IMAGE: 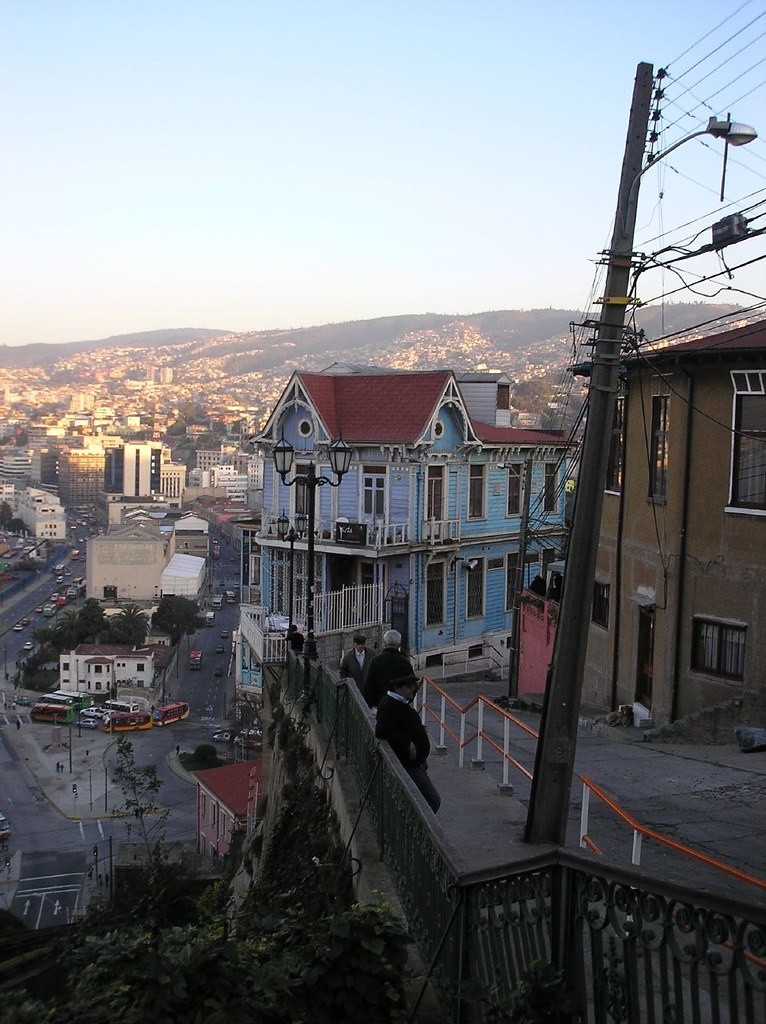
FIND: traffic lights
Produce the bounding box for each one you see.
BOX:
[72,784,77,793]
[92,846,98,857]
[4,857,10,868]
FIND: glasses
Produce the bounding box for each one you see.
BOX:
[354,643,365,645]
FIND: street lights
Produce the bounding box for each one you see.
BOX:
[272,427,353,659]
[78,690,84,738]
[525,116,758,847]
[497,458,532,700]
[276,508,307,629]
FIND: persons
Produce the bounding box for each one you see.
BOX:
[375,666,442,814]
[531,573,547,598]
[56,762,64,773]
[4,700,16,710]
[285,625,305,657]
[16,720,20,730]
[176,745,180,755]
[339,636,377,682]
[361,630,414,708]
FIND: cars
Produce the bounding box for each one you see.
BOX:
[0,813,12,839]
[3,538,35,558]
[213,727,259,747]
[13,564,85,650]
[70,514,104,562]
[77,719,98,729]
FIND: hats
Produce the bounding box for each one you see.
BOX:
[387,668,420,687]
[353,635,366,642]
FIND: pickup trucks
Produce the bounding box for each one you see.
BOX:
[80,708,106,718]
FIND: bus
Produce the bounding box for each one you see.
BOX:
[99,700,140,714]
[152,702,190,726]
[103,712,152,731]
[30,690,94,723]
[188,581,239,676]
